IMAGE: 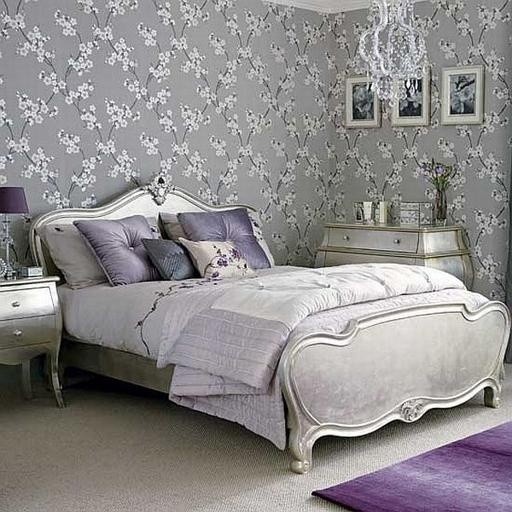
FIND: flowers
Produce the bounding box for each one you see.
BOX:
[420,159,460,218]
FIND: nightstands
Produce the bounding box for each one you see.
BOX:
[0,273,66,411]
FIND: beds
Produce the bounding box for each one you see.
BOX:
[30,172,512,475]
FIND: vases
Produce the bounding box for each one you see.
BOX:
[435,189,448,224]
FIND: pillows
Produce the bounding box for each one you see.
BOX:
[178,209,270,268]
[159,211,274,264]
[142,237,192,280]
[178,234,253,278]
[74,215,158,285]
[37,217,162,289]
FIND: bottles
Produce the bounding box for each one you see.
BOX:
[354,202,363,223]
[374,204,380,222]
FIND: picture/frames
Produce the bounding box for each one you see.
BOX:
[442,65,484,124]
[344,76,381,128]
[392,67,430,128]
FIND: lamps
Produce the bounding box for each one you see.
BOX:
[0,188,29,275]
[356,0,427,118]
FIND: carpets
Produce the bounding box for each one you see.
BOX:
[311,420,512,512]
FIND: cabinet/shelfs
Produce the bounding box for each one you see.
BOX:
[315,222,473,290]
